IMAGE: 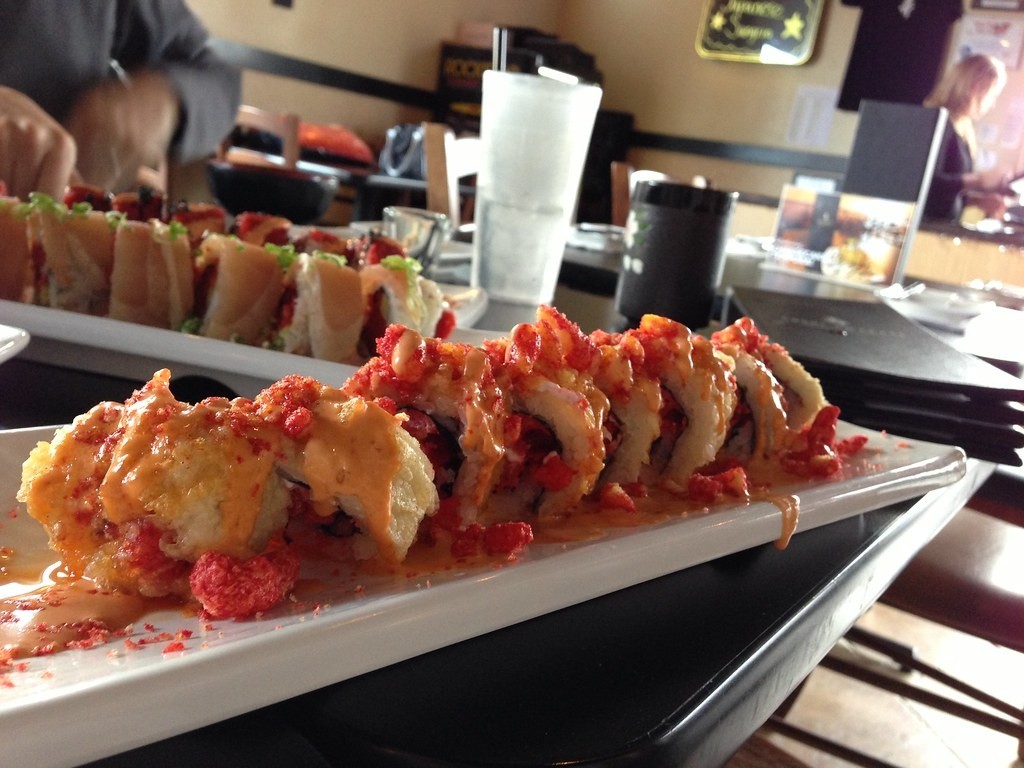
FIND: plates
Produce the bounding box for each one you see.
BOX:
[893,301,984,325]
[0,418,971,768]
[0,298,362,399]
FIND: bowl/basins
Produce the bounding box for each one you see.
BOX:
[205,160,340,224]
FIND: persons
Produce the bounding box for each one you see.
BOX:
[918,54,1024,226]
[1,0,243,213]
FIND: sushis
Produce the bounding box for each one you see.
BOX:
[18,300,824,604]
[1,184,455,367]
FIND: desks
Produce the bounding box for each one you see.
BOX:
[211,146,1024,504]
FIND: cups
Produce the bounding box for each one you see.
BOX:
[470,69,603,305]
[614,179,740,330]
[381,205,454,282]
